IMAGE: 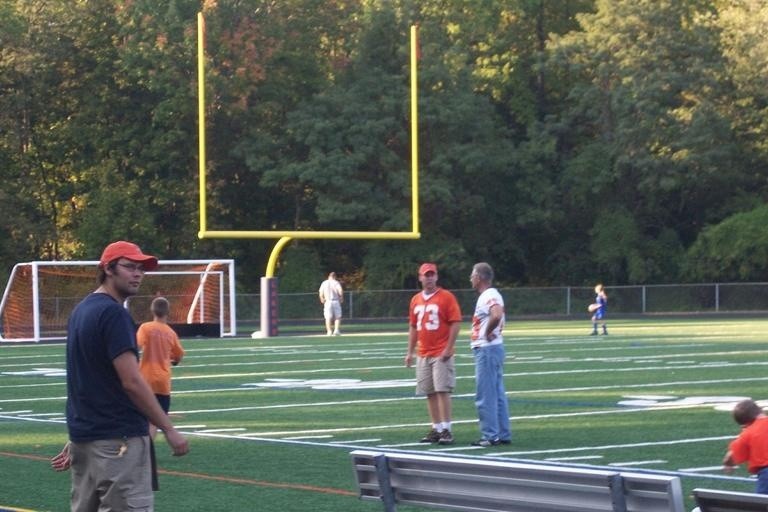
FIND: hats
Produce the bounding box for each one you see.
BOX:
[418,263,437,275]
[101,241,158,271]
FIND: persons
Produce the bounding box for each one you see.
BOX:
[319,272,344,335]
[51,240,189,512]
[589,285,608,335]
[136,297,186,440]
[470,263,511,446]
[405,263,462,443]
[723,401,768,494]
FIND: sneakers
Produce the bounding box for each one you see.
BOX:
[439,429,454,442]
[472,438,512,446]
[420,429,441,442]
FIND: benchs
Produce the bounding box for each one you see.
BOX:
[691,486,767,512]
[346,446,686,512]
